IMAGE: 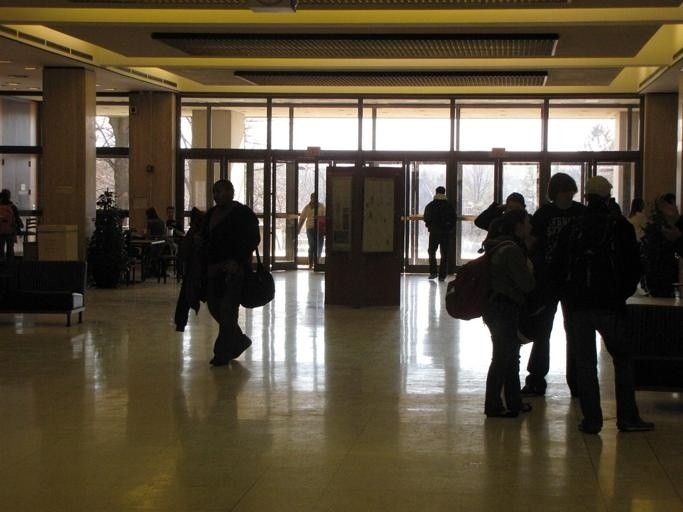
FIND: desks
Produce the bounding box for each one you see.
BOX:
[121,237,165,289]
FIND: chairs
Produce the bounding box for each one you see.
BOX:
[159,239,188,284]
[21,217,39,256]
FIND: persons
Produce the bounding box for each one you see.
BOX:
[192,179,261,366]
[623,197,652,296]
[516,173,587,398]
[556,175,657,433]
[474,192,534,261]
[298,192,326,268]
[141,208,165,258]
[654,193,682,296]
[0,189,23,257]
[423,187,457,281]
[477,209,533,418]
[165,205,183,280]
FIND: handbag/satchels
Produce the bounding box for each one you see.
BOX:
[239,242,275,310]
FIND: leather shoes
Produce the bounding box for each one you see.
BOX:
[428,273,437,279]
[439,276,445,281]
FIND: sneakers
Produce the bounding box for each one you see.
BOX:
[519,386,545,398]
[577,422,604,434]
[617,418,656,432]
[208,333,251,366]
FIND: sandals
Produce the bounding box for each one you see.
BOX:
[485,399,534,418]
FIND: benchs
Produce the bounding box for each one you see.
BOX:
[0,257,90,328]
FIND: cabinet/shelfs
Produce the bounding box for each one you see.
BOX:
[37,223,79,262]
[614,290,683,395]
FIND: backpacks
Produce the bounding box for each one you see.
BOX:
[445,240,517,321]
[0,203,15,232]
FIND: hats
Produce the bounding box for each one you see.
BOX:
[583,176,612,197]
